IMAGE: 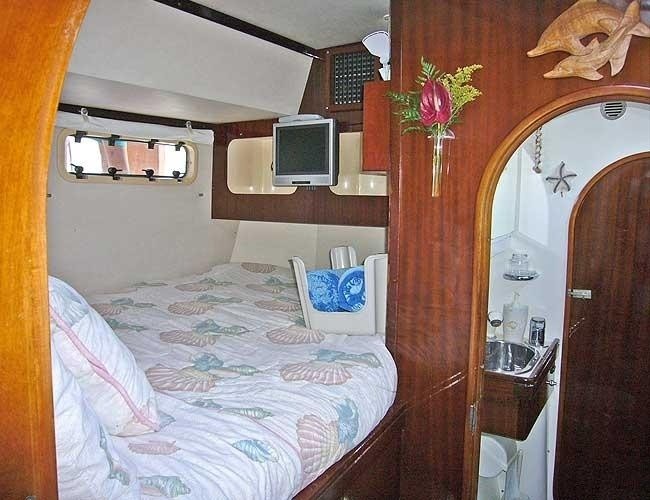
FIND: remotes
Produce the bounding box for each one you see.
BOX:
[279,114,324,122]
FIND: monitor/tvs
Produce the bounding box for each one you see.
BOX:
[273,118,339,186]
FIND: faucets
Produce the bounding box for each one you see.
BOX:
[487,310,504,339]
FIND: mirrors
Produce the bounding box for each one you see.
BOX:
[63,135,191,183]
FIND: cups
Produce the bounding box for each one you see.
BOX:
[510,253,530,276]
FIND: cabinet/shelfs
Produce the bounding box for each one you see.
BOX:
[483,362,556,441]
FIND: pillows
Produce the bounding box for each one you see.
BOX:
[48,274,173,438]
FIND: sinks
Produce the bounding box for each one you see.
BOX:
[483,339,541,374]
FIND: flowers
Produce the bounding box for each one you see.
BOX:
[382,59,482,196]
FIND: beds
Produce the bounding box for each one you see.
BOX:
[53,259,397,492]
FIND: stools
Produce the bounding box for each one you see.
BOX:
[290,245,388,336]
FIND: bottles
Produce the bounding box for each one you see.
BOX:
[530,316,546,347]
[501,290,530,344]
